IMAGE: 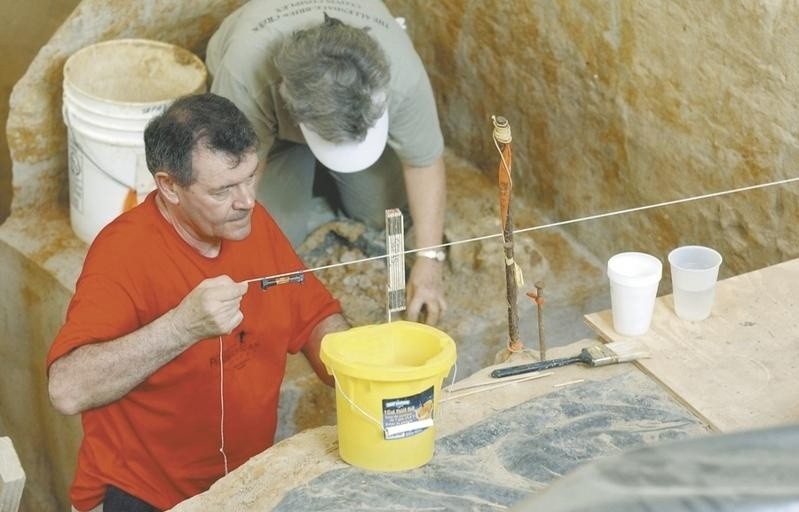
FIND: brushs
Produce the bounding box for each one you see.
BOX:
[491,340,652,378]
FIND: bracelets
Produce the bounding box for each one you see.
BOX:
[416,249,448,263]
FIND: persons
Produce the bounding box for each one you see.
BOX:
[204,1,452,329]
[45,90,353,511]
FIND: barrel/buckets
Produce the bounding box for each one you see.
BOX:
[62,39,209,245]
[318,319,458,471]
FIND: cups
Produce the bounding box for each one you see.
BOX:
[668,245,723,322]
[606,252,663,336]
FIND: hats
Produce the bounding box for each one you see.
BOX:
[298,87,390,175]
